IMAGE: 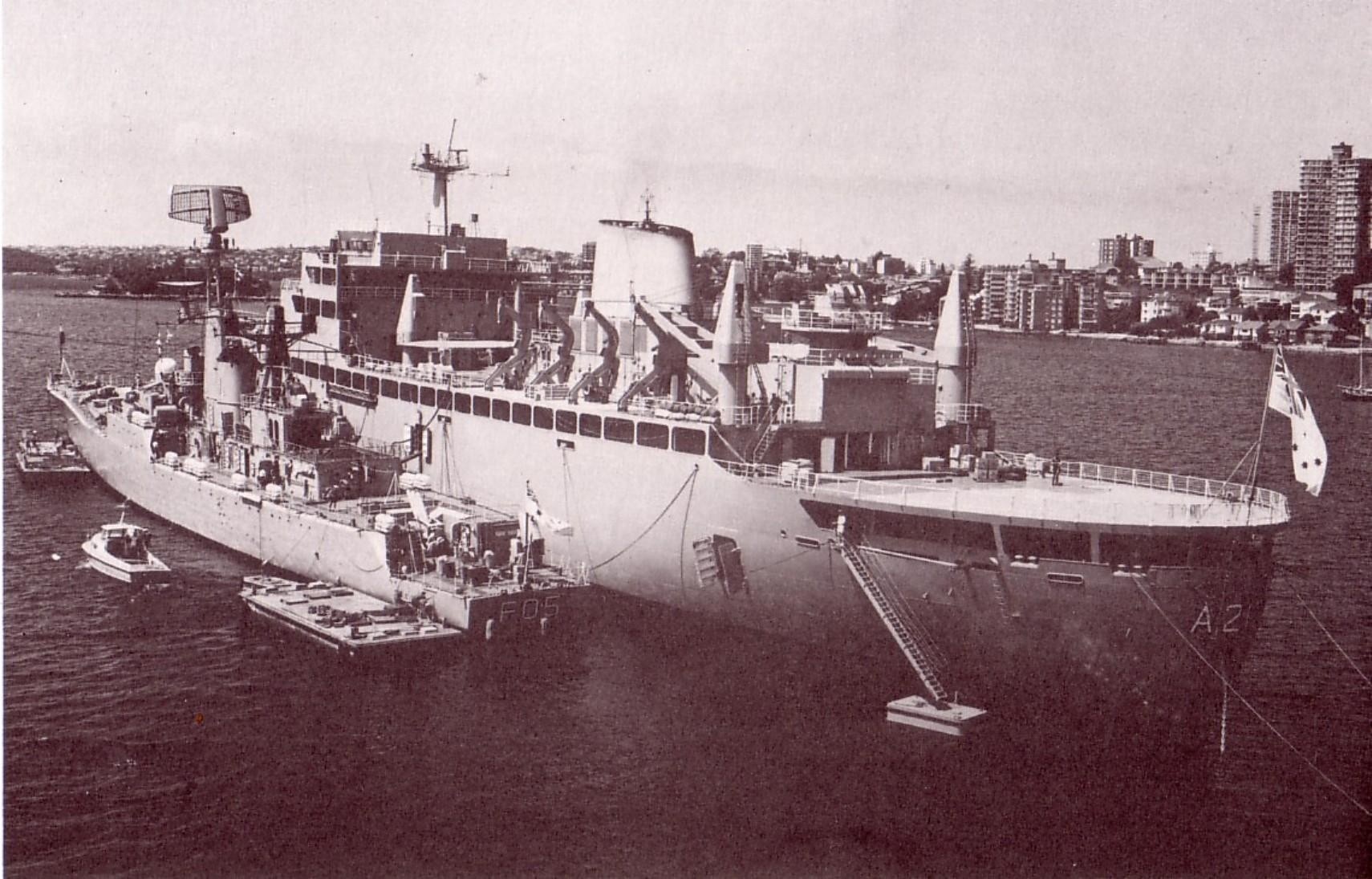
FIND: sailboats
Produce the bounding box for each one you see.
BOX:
[1335,325,1372,400]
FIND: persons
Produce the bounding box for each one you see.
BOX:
[257,451,293,490]
[121,526,153,563]
[1051,451,1061,485]
[130,372,143,392]
[325,485,340,508]
[746,392,784,422]
[23,426,67,458]
[504,370,522,392]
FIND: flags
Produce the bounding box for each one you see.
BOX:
[526,488,544,520]
[1266,343,1328,497]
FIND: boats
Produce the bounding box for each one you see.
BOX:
[14,427,91,487]
[45,184,599,641]
[239,573,465,661]
[82,499,173,587]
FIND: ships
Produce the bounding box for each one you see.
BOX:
[242,117,1294,746]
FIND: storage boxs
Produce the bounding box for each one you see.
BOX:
[922,443,997,481]
